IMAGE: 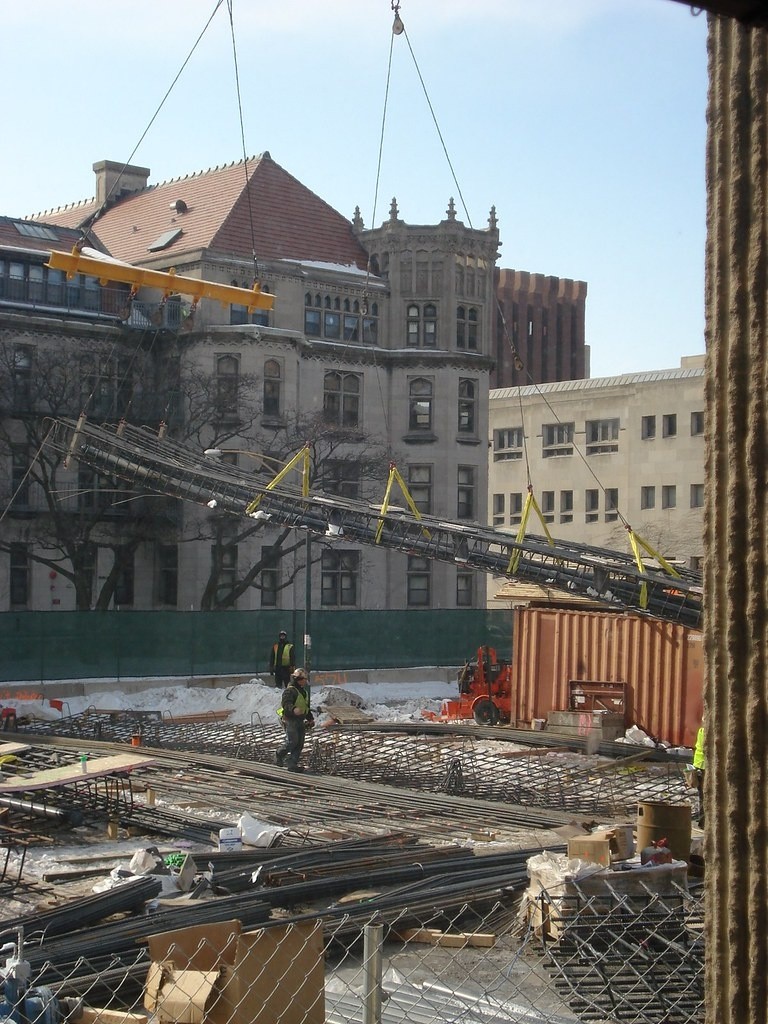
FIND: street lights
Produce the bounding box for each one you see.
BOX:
[204,439,314,713]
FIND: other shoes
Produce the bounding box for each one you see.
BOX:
[276,751,283,767]
[288,765,303,773]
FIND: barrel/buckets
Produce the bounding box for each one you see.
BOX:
[634,800,692,874]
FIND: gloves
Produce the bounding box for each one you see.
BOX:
[270,667,275,675]
[289,665,295,674]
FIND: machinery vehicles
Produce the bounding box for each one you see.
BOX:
[418,642,514,730]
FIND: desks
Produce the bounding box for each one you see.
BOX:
[0,754,156,824]
[0,742,32,753]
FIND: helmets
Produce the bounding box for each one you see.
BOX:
[290,668,309,679]
[278,631,288,636]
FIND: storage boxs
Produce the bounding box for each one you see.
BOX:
[532,718,546,730]
[146,919,325,1024]
[548,679,626,742]
[527,824,686,941]
[161,852,197,893]
[210,827,242,852]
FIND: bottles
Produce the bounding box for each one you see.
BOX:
[81,757,88,774]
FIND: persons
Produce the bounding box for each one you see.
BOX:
[275,668,315,772]
[270,631,296,688]
[692,728,704,822]
